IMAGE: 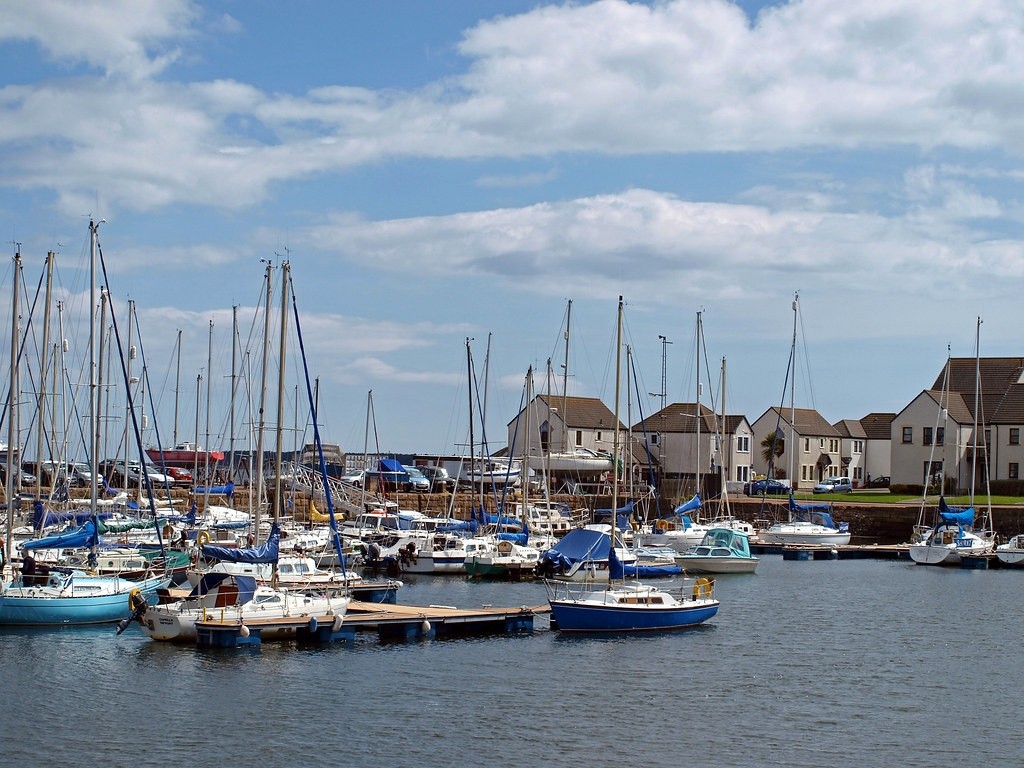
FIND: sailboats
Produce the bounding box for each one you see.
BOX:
[1,215,1023,651]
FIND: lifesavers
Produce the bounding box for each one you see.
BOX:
[198,531,210,545]
[656,519,668,530]
[693,577,712,599]
[129,588,141,611]
